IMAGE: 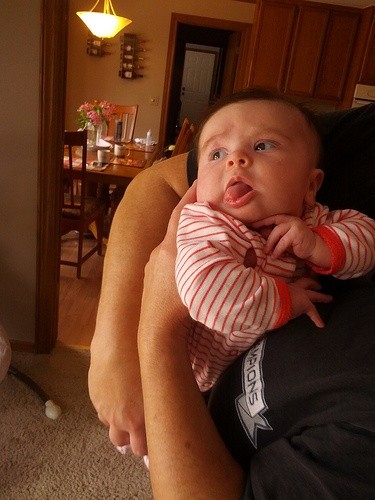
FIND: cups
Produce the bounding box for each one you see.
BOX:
[114,144,130,158]
[97,150,110,162]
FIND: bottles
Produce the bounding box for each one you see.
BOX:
[146,129,152,146]
[86,122,95,151]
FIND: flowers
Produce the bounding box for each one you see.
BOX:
[76,99,116,131]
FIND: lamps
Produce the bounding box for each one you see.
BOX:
[76,0,133,39]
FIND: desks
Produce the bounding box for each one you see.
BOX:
[61,137,162,236]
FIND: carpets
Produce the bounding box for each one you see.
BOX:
[0,343,153,500]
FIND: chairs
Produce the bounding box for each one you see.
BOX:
[104,103,138,140]
[169,119,196,156]
[60,130,108,279]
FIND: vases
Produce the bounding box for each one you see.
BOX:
[87,121,108,150]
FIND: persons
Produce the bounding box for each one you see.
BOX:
[108,88,375,471]
[87,100,375,500]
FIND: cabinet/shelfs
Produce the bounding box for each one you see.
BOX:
[119,33,137,78]
[87,31,104,57]
[243,0,375,109]
